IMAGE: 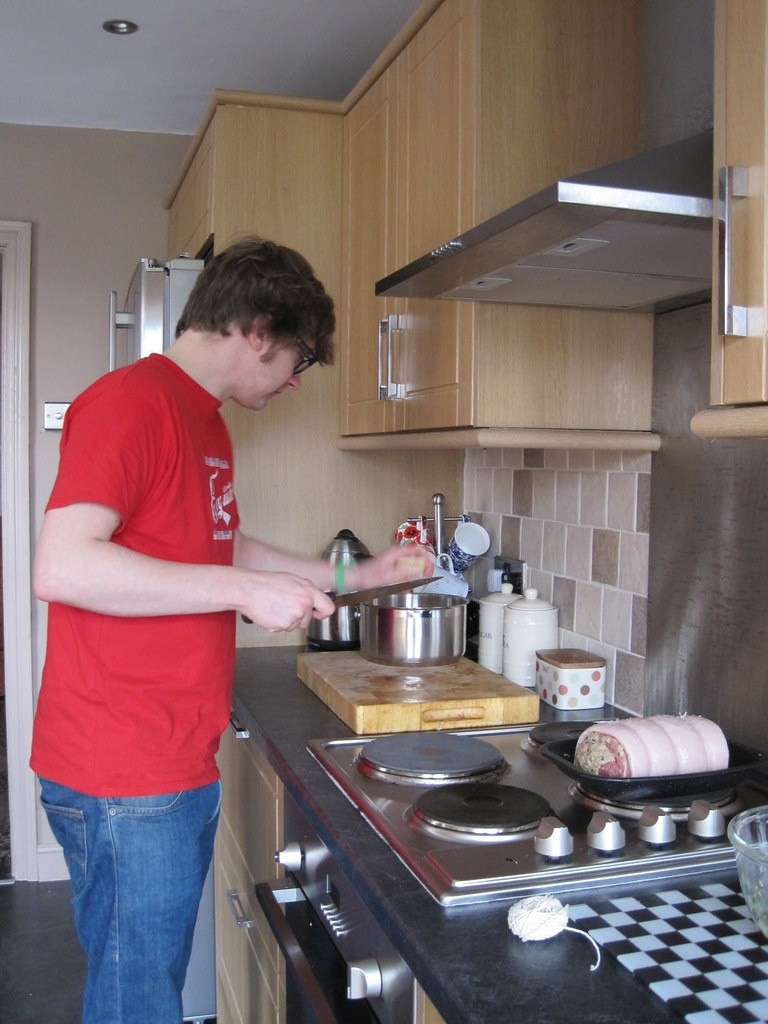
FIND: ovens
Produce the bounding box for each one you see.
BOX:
[256,783,420,1024]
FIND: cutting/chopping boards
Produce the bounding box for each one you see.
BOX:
[295,651,539,735]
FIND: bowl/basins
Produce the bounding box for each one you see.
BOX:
[726,805,768,940]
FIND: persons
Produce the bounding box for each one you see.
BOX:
[30,238,434,1024]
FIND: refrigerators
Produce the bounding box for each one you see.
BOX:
[107,252,215,1024]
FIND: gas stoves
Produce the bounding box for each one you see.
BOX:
[305,713,768,907]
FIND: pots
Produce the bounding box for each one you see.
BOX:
[351,592,471,667]
[541,739,768,801]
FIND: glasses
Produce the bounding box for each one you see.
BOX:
[288,331,319,375]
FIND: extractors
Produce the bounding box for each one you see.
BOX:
[375,128,725,315]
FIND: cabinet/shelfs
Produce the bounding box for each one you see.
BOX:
[691,0,768,441]
[215,705,285,1023]
[163,1,664,452]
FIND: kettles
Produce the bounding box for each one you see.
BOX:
[306,530,376,651]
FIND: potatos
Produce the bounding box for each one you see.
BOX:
[393,555,426,577]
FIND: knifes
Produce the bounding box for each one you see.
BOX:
[241,575,444,626]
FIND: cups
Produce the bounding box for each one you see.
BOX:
[503,588,559,686]
[478,582,524,674]
[445,515,491,572]
[395,514,436,556]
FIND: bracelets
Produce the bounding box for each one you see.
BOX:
[336,564,345,592]
